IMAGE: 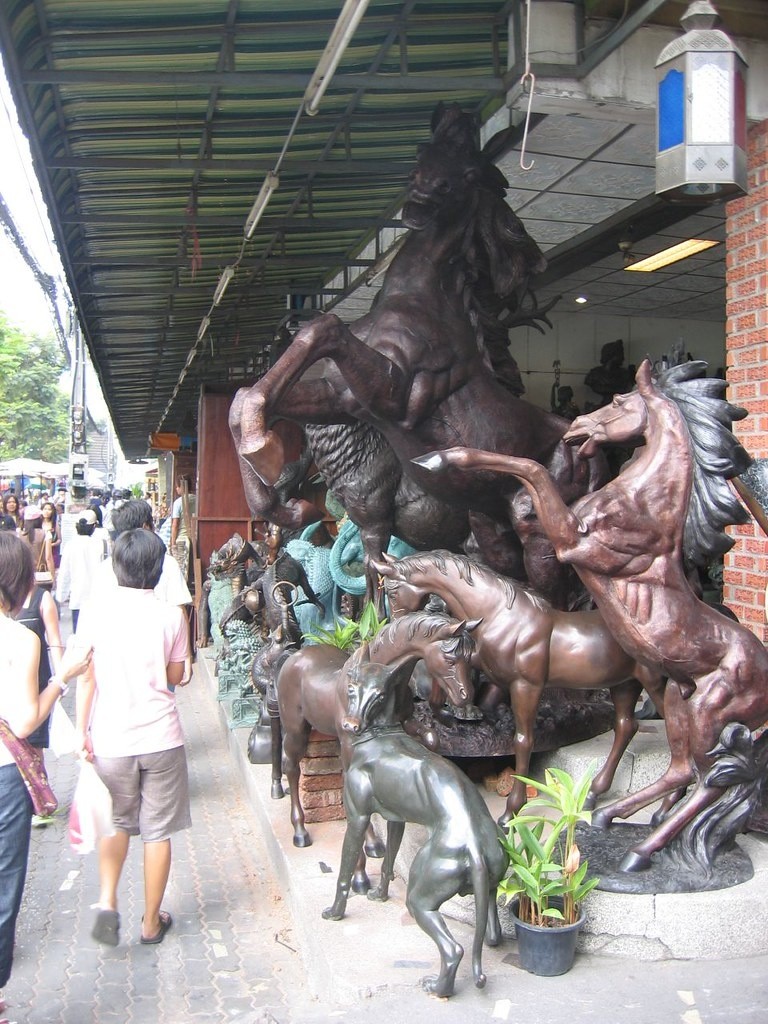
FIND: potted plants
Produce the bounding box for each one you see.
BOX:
[492,756,600,974]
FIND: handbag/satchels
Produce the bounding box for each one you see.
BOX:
[9,737,58,817]
[33,563,54,585]
[69,749,116,855]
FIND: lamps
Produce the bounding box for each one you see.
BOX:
[654,0,751,208]
[155,369,187,433]
[303,0,371,116]
[186,349,197,366]
[197,316,211,340]
[212,265,238,307]
[242,170,281,241]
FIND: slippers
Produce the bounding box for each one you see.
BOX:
[140,909,171,944]
[92,911,120,947]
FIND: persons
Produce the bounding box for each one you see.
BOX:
[0,472,197,988]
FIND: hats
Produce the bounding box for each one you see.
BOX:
[76,509,100,525]
[24,505,44,520]
[115,491,123,497]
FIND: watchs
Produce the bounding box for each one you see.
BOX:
[48,675,70,697]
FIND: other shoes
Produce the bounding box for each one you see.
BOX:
[0,990,5,1013]
[31,814,55,827]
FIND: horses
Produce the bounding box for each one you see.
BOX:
[207,97,768,998]
